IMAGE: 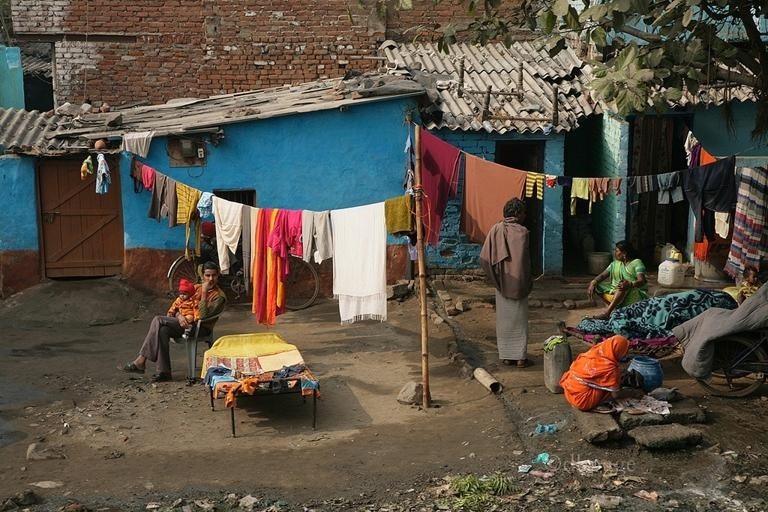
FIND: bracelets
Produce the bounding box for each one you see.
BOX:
[629,281,634,287]
[590,280,598,287]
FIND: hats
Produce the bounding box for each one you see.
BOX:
[179,279,195,296]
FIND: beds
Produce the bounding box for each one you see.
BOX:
[558,321,679,367]
[204,333,320,437]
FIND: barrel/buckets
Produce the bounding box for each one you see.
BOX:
[543,341,571,394]
[655,241,685,288]
[587,251,611,275]
[627,356,664,395]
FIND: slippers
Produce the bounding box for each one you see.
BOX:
[117,362,144,374]
[592,401,617,414]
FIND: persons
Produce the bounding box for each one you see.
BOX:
[482,197,536,369]
[112,261,228,381]
[166,279,201,339]
[737,262,762,306]
[587,240,649,320]
[557,335,646,413]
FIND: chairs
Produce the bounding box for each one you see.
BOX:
[170,308,225,380]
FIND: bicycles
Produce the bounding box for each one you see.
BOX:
[687,326,767,399]
[166,221,320,311]
